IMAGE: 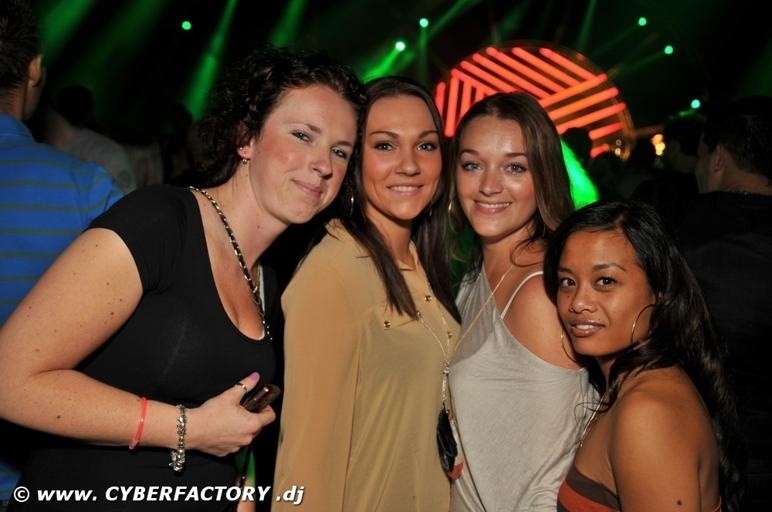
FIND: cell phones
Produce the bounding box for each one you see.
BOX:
[243,383,281,414]
